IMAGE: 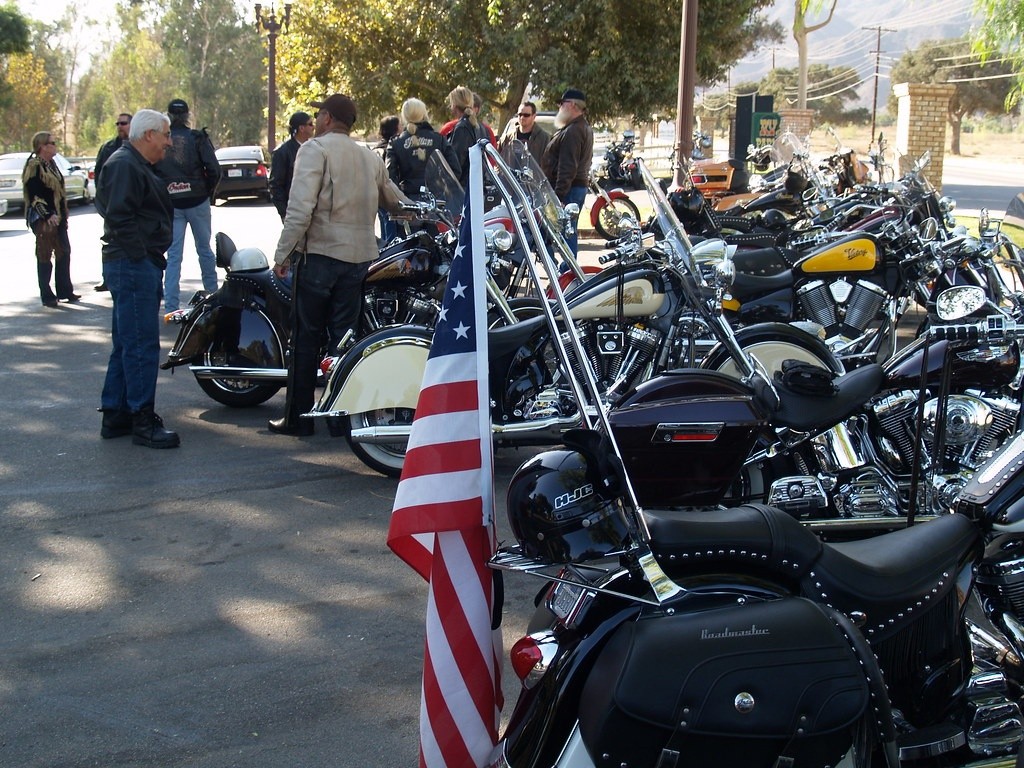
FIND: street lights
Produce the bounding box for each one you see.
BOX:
[252,1,294,165]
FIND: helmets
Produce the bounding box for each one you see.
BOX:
[667,187,704,228]
[623,130,635,137]
[507,449,627,562]
[701,139,711,148]
[230,247,269,273]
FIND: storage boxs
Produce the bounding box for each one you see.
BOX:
[589,367,764,509]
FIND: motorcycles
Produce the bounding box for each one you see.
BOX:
[668,129,712,183]
[602,129,644,187]
[156,146,468,410]
[473,122,1024,768]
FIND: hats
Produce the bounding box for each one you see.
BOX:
[554,90,585,104]
[310,93,357,124]
[168,99,188,114]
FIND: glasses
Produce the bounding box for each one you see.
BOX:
[560,101,576,106]
[115,122,130,126]
[45,142,55,145]
[313,112,327,118]
[518,113,533,117]
[304,122,314,126]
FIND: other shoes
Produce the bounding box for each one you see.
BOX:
[58,294,81,301]
[43,301,56,307]
[95,284,109,291]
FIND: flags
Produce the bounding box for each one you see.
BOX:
[385,194,509,768]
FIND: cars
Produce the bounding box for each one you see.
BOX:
[0,152,96,217]
[206,146,273,206]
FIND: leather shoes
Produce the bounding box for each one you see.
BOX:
[101,410,134,437]
[328,417,347,436]
[268,417,314,435]
[132,409,180,448]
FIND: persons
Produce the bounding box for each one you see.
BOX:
[93,110,181,448]
[541,88,594,274]
[267,112,316,225]
[448,85,491,184]
[438,92,496,167]
[154,98,221,315]
[384,98,462,267]
[269,94,417,435]
[92,113,132,290]
[21,131,81,308]
[498,100,549,207]
[372,116,402,242]
